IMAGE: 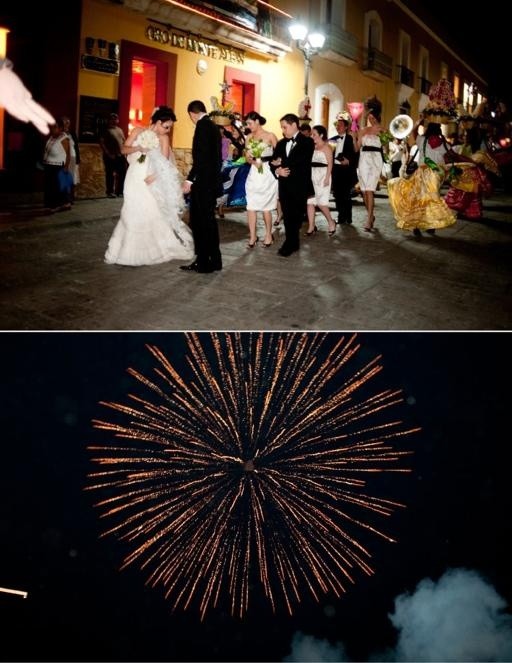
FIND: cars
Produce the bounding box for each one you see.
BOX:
[487,137,511,151]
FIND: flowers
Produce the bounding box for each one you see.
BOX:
[374,126,394,162]
[247,135,272,173]
[137,128,161,162]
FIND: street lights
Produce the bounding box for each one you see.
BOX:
[287,12,328,129]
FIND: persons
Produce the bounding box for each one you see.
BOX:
[44,120,81,218]
[59,116,81,161]
[102,96,494,269]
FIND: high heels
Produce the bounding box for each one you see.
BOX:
[249,215,317,256]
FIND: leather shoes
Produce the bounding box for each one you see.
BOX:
[180,255,222,273]
[328,216,375,237]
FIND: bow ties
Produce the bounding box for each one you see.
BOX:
[337,136,343,139]
[286,137,295,143]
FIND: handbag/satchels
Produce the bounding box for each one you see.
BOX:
[406,162,418,175]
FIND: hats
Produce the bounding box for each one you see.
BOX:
[460,121,473,129]
[479,123,488,128]
[212,116,232,126]
[424,114,452,124]
